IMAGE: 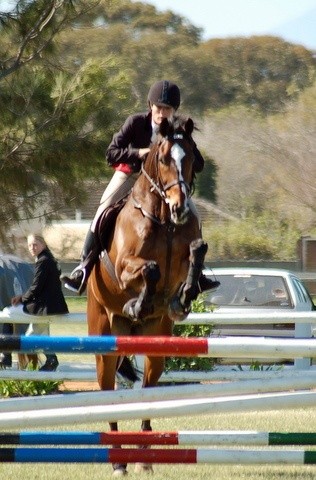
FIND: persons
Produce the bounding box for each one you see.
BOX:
[61,86,221,294]
[11,231,70,373]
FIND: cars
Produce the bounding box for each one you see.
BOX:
[131,266,316,370]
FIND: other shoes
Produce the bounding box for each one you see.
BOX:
[60,272,86,296]
[39,354,58,372]
[0,353,12,367]
[195,275,220,291]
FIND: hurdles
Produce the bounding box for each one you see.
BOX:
[0,310,316,466]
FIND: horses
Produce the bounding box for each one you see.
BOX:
[87,117,208,477]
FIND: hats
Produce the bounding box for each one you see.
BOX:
[147,80,181,112]
